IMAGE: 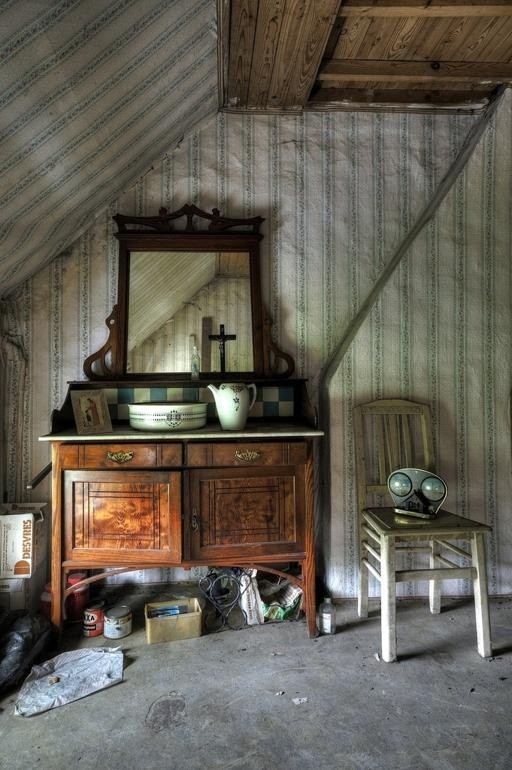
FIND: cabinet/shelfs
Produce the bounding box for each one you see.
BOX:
[38,427,326,642]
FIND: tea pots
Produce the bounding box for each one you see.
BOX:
[208,382,258,433]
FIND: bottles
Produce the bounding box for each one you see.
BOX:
[318,596,336,635]
[39,571,92,624]
[191,347,201,381]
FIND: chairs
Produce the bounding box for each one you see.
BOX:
[348,397,495,664]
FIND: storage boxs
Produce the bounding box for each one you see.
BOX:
[0,500,49,581]
[1,562,47,618]
[145,593,205,646]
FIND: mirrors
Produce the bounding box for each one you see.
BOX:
[80,203,295,381]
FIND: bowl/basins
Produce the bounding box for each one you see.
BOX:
[126,400,211,431]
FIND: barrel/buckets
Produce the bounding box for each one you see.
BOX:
[40,583,52,620]
[82,600,106,638]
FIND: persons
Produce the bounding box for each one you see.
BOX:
[85,398,100,426]
